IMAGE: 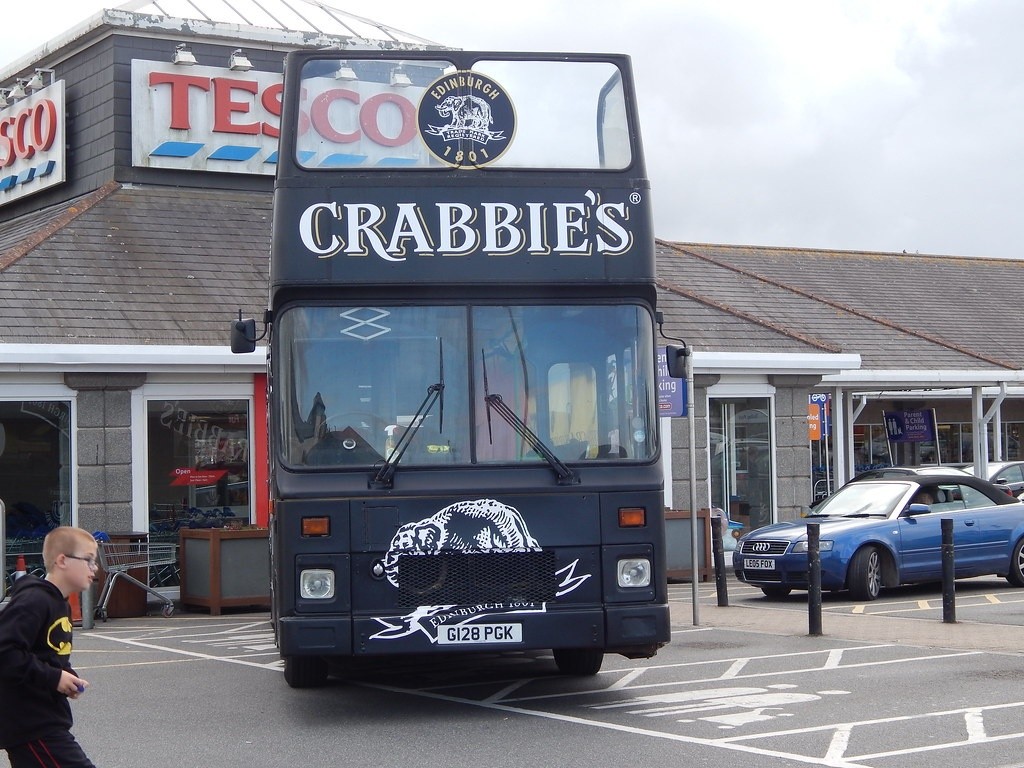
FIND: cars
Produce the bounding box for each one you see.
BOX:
[962,461,1024,498]
[709,409,1022,528]
[807,465,1012,510]
[733,466,1024,602]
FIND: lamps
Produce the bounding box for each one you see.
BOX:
[228,48,254,72]
[171,41,198,66]
[0,68,56,107]
[390,66,413,88]
[335,59,357,82]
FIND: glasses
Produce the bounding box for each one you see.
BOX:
[64,553,98,565]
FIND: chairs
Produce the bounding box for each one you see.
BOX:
[932,489,946,504]
[942,489,953,502]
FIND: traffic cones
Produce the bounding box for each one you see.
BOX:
[16,553,27,583]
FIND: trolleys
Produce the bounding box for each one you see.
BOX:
[6,506,241,594]
[92,531,179,623]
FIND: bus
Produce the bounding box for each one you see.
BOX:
[226,45,693,689]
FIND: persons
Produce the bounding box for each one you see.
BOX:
[0,526,98,768]
[917,493,933,508]
